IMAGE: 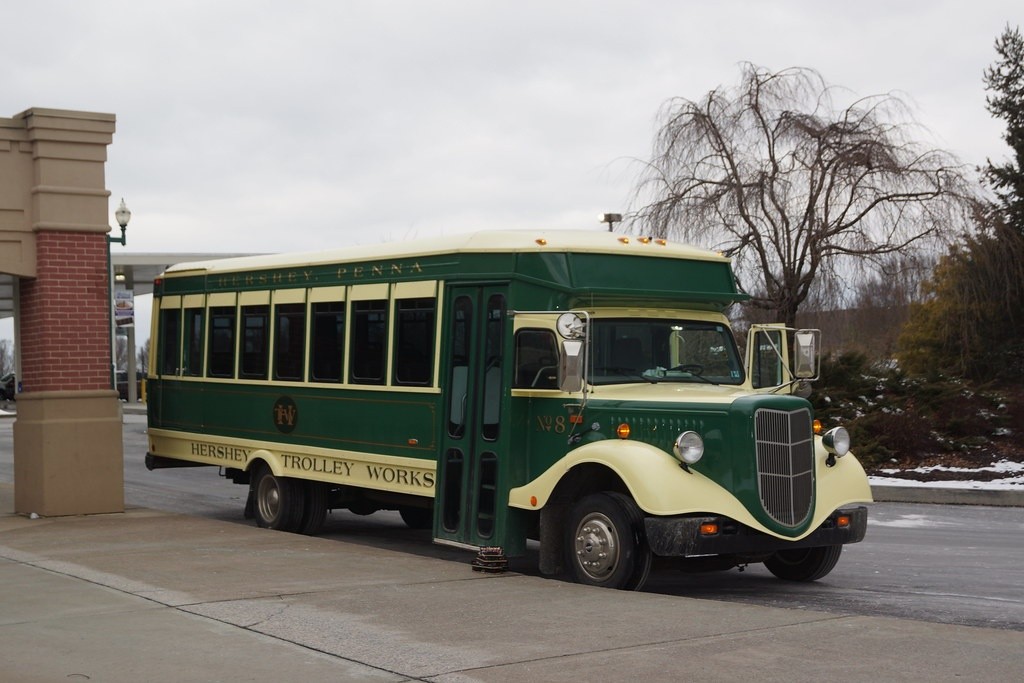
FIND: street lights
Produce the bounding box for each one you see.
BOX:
[601,214,622,231]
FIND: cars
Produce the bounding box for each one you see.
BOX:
[116,371,142,403]
[0,373,15,399]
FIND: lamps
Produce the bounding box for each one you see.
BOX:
[110,196,132,246]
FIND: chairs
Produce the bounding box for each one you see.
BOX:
[611,337,641,374]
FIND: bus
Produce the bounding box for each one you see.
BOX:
[142,231,875,590]
